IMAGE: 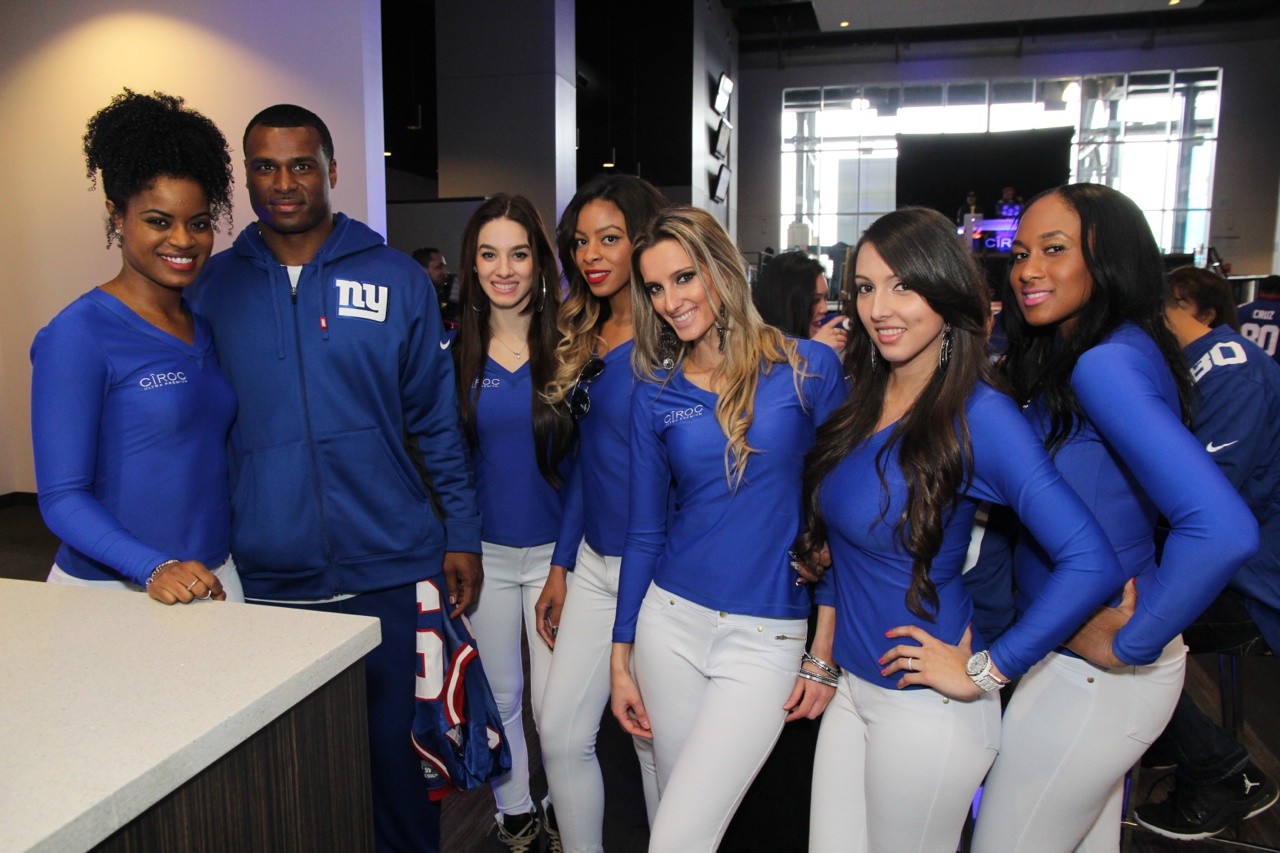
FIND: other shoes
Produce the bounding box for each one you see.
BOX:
[539,800,567,853]
[494,805,541,853]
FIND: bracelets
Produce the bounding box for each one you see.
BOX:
[802,650,840,679]
[145,559,179,587]
[799,669,839,688]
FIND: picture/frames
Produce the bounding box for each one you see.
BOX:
[712,71,733,204]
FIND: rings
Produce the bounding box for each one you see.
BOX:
[186,578,199,591]
[909,657,912,671]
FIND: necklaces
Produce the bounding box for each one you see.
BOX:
[489,331,528,359]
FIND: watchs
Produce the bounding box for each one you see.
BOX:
[965,650,1011,694]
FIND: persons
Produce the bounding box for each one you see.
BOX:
[412,173,1280,853]
[180,104,486,853]
[30,87,245,605]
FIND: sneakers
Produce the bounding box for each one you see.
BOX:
[1135,769,1279,840]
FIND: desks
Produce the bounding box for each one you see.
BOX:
[0,577,382,853]
